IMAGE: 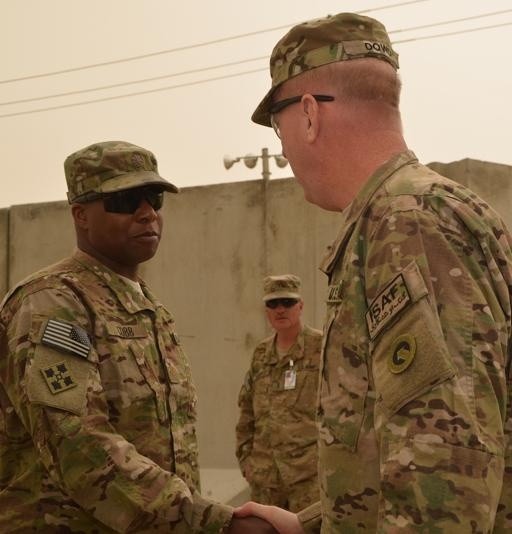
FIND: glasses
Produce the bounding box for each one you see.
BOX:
[104,184,164,214]
[268,95,335,139]
[266,298,298,309]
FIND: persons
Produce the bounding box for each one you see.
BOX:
[236,275,324,513]
[252,12,511,534]
[0,140,278,534]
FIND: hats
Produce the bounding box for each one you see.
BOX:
[262,274,302,302]
[64,141,179,205]
[251,12,400,128]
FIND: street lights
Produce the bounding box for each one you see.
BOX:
[223,146,290,178]
